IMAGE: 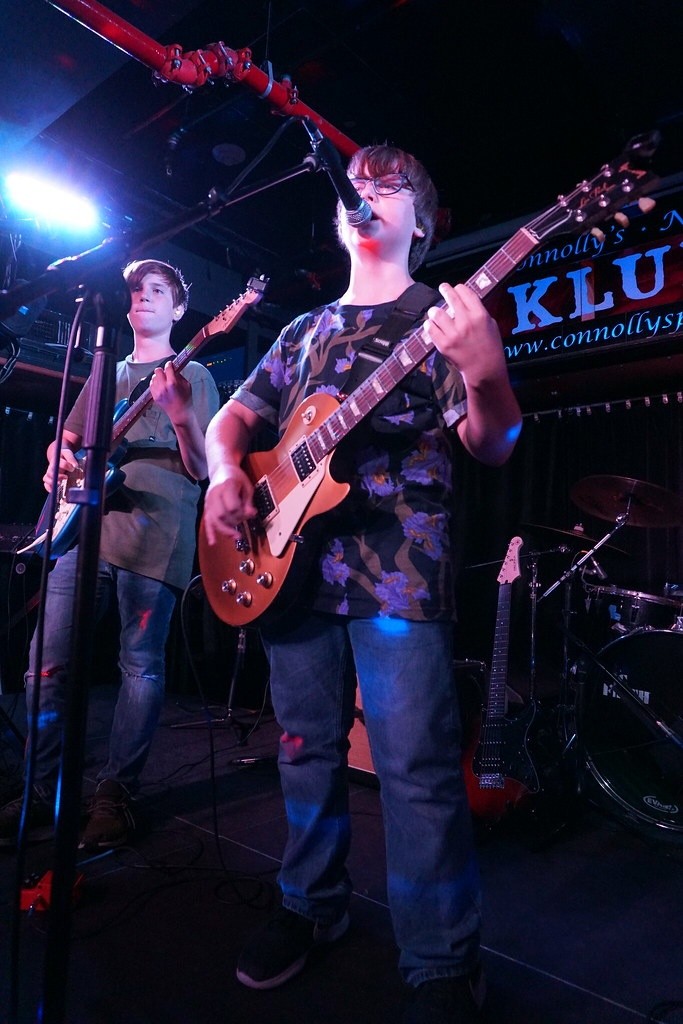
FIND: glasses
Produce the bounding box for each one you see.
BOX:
[347,171,419,197]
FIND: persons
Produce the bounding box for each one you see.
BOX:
[0,260,220,853]
[567,550,617,684]
[206,146,522,1024]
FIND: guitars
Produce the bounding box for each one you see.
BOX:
[197,153,658,628]
[31,274,272,561]
[454,533,545,841]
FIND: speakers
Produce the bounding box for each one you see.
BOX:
[344,660,486,792]
[0,549,53,696]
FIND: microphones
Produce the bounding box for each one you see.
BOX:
[590,556,608,581]
[301,115,372,228]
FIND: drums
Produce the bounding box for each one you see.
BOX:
[560,623,682,833]
[580,580,679,633]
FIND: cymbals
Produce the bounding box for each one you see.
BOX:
[521,521,631,557]
[570,473,682,529]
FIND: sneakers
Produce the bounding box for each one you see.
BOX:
[0,783,60,838]
[405,977,477,1024]
[234,899,349,990]
[79,779,138,849]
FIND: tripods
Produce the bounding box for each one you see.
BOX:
[166,628,259,748]
